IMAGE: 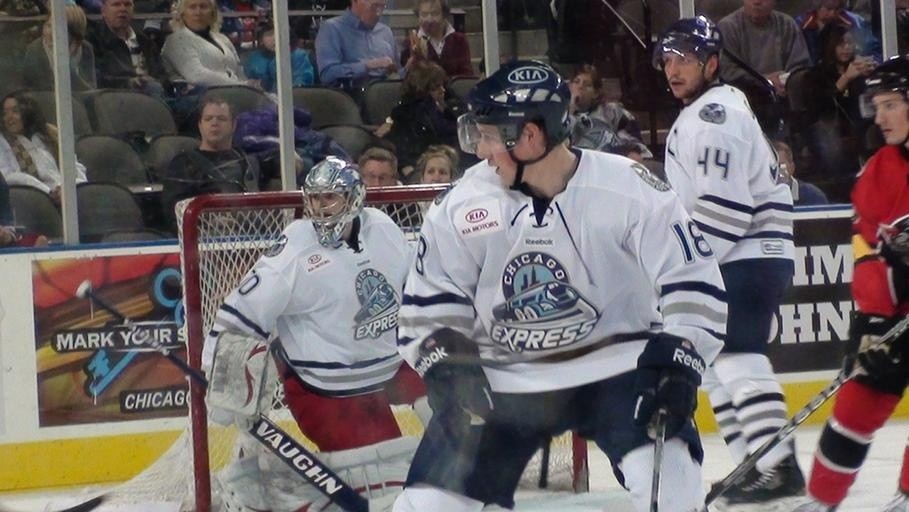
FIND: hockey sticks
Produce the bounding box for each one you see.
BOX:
[74,280,401,512]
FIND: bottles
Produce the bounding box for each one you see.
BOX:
[779,165,791,188]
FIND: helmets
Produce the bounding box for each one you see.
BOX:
[651,14,724,83]
[253,14,275,34]
[301,156,368,248]
[859,55,907,118]
[454,58,575,159]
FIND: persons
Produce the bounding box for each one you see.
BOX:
[400,58,727,511]
[794,49,909,512]
[200,157,434,512]
[2,0,475,248]
[658,17,807,512]
[568,1,909,202]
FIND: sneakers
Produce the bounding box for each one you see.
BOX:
[718,454,809,512]
[707,452,769,508]
[876,493,909,512]
[763,493,838,512]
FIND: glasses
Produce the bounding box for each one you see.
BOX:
[360,171,399,185]
[362,0,389,12]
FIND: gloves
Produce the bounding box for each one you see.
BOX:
[412,327,500,451]
[632,335,709,444]
[838,318,893,382]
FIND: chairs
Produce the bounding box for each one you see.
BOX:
[782,66,819,119]
